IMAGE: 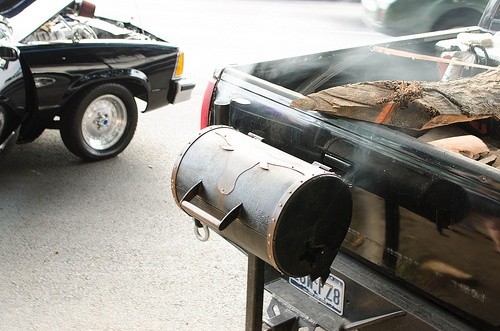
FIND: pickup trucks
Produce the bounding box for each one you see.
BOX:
[172,0,500,331]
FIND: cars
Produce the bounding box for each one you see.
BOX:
[360,0,489,37]
[0,0,196,162]
